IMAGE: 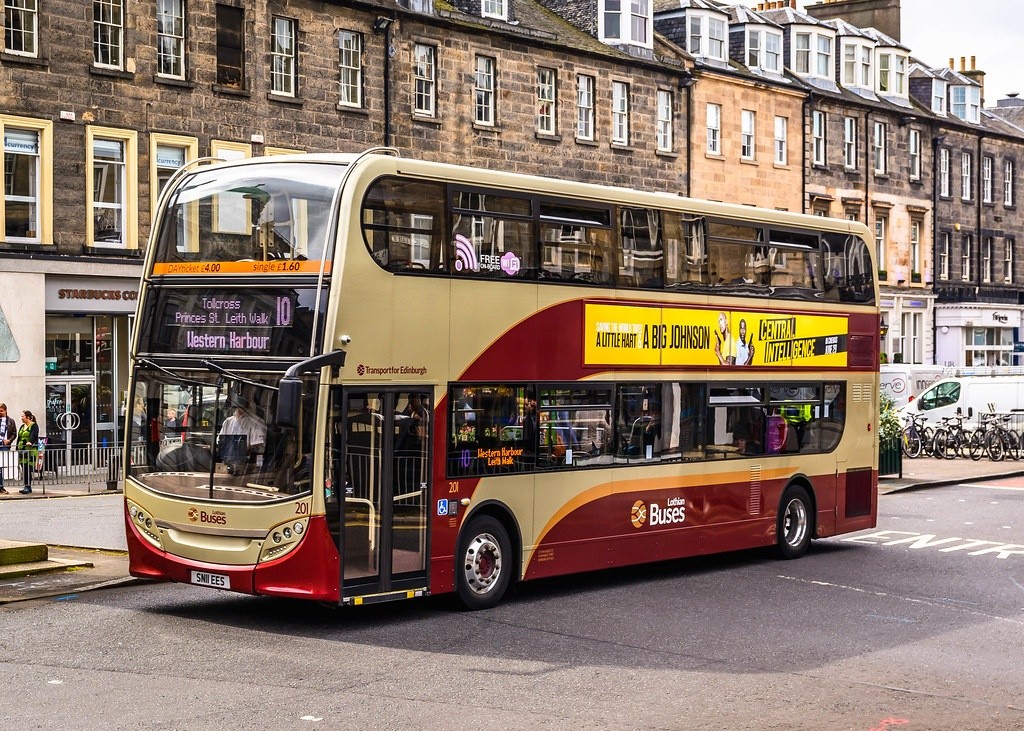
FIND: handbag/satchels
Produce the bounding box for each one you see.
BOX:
[551,435,566,456]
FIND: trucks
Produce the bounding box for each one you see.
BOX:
[880,376,1024,449]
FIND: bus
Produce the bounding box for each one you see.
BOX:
[123,147,880,608]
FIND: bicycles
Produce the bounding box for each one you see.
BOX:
[898,411,1023,461]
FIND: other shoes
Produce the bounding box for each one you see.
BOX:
[19,486,32,494]
[1,487,9,494]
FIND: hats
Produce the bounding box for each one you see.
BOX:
[242,189,271,204]
[231,396,250,408]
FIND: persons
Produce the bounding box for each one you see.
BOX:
[16,411,39,494]
[216,397,266,471]
[571,407,612,459]
[732,430,750,453]
[0,403,17,494]
[736,319,755,366]
[714,312,737,366]
[165,409,181,427]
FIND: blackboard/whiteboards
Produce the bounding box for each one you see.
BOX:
[37,436,58,482]
